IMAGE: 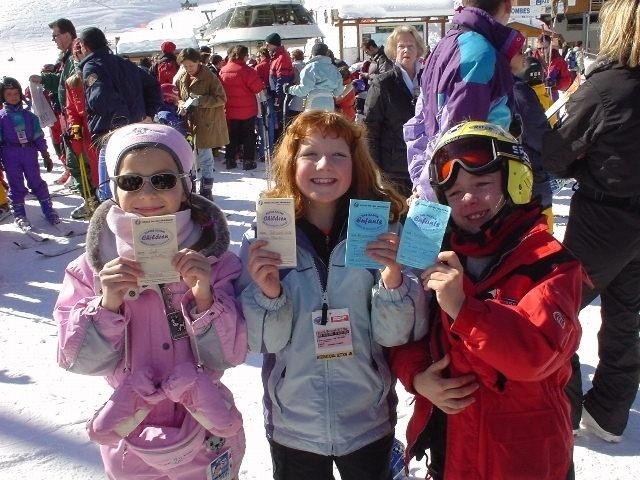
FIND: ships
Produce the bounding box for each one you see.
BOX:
[194,0,326,60]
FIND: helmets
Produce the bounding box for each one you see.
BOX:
[160,83,180,104]
[427,119,537,214]
[519,56,544,85]
[352,78,366,92]
[0,76,24,105]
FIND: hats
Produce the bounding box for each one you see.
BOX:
[161,41,177,55]
[72,36,82,54]
[504,27,526,63]
[104,121,195,207]
[80,26,107,47]
[264,32,281,47]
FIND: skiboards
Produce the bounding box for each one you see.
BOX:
[14,213,89,258]
[16,217,76,242]
[47,178,74,192]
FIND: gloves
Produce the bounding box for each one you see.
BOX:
[69,123,83,142]
[42,157,54,172]
[546,76,559,88]
[84,367,169,449]
[184,97,197,111]
[159,362,245,436]
[282,82,293,94]
[273,98,281,111]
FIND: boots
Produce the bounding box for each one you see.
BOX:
[10,203,32,231]
[53,155,71,184]
[199,176,214,202]
[62,174,76,189]
[40,198,61,226]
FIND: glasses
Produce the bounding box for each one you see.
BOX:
[51,31,66,39]
[364,48,371,54]
[105,173,191,194]
[514,46,526,56]
[537,45,549,52]
[264,43,272,48]
[244,53,250,57]
[428,134,532,193]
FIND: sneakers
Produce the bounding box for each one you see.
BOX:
[70,195,97,220]
[571,427,581,441]
[225,158,239,170]
[583,397,625,445]
[243,159,258,170]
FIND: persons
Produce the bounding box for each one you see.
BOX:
[232,109,431,479]
[388,121,595,480]
[542,0,640,444]
[53,122,248,480]
[0,1,583,234]
